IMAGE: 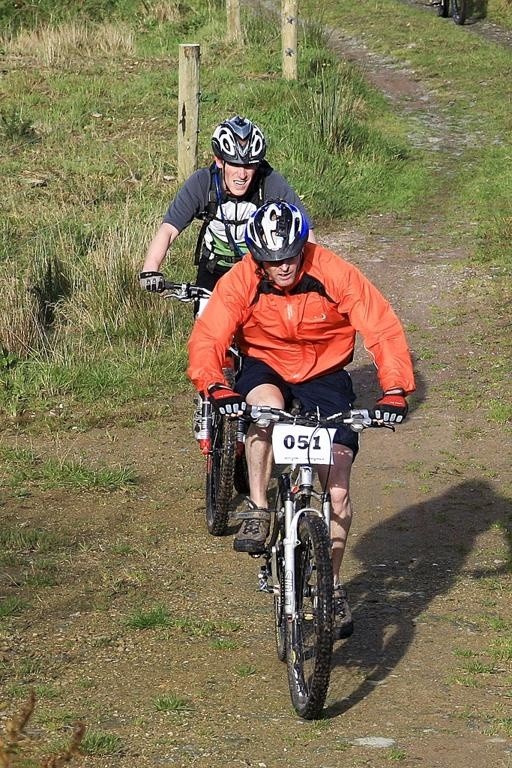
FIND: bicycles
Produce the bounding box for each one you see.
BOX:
[154,279,241,538]
[219,399,394,720]
[425,0,467,25]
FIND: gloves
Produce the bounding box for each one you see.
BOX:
[207,383,247,417]
[139,271,165,293]
[370,386,408,424]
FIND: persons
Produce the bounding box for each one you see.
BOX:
[186,196,418,644]
[138,114,318,444]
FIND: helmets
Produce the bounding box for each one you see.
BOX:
[244,199,309,262]
[210,115,267,166]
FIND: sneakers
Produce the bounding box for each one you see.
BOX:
[194,403,216,441]
[232,507,271,553]
[330,584,354,641]
